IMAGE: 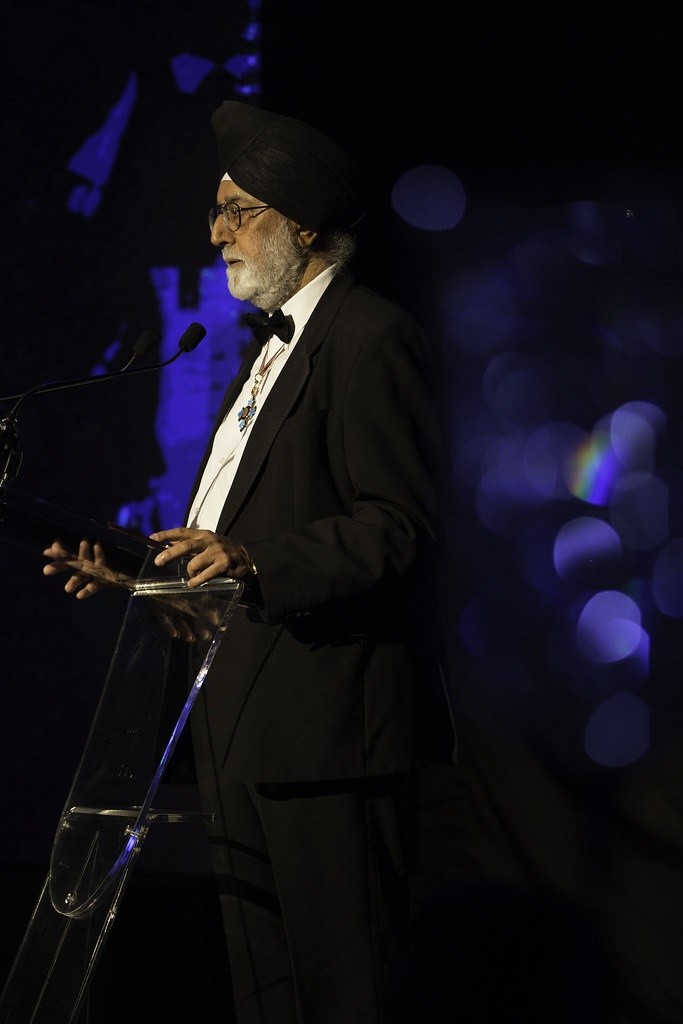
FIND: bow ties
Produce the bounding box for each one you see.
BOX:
[240,308,291,346]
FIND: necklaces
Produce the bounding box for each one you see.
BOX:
[238,337,286,433]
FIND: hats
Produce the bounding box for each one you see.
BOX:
[214,96,372,235]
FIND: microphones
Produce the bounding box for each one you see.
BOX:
[5,323,205,425]
[0,328,156,402]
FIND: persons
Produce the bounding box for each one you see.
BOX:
[43,101,456,1024]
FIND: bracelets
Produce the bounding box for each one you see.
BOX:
[253,564,257,574]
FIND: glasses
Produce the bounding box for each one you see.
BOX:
[210,199,271,232]
